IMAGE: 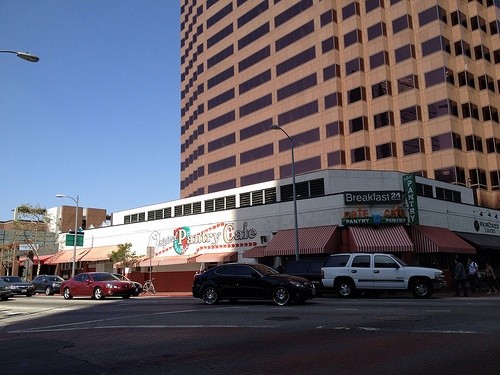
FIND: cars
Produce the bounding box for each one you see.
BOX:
[0,279,14,303]
[30,274,65,295]
[0,276,36,298]
[190,262,318,306]
[60,272,137,300]
[281,258,326,287]
[113,273,143,296]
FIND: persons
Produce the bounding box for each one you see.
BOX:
[485,263,498,294]
[448,254,468,297]
[467,257,479,291]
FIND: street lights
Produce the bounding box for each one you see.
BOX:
[55,194,80,277]
[269,123,300,260]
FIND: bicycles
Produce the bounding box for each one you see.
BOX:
[148,278,156,294]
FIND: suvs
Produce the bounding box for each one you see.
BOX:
[320,253,449,298]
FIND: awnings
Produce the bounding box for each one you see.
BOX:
[348,224,414,252]
[263,225,339,256]
[455,232,500,250]
[242,246,266,257]
[409,224,476,255]
[196,252,238,263]
[18,255,56,266]
[113,254,202,269]
[44,245,121,265]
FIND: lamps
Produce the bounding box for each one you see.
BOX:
[473,208,484,217]
[482,209,491,217]
[490,212,498,219]
